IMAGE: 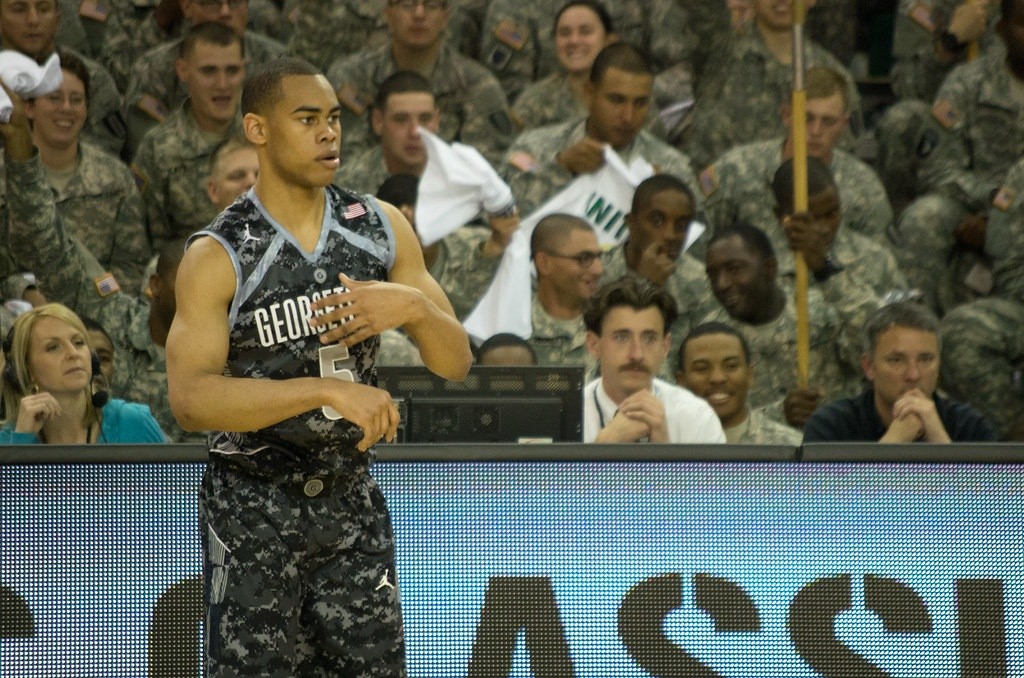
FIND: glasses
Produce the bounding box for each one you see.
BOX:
[548,251,604,268]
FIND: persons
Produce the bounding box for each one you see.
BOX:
[164,56,475,678]
[0,0,1024,447]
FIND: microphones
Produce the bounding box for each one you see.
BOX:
[92,372,109,409]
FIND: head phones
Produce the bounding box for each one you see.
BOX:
[1,320,102,393]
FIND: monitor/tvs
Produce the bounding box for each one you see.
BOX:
[371,363,588,443]
